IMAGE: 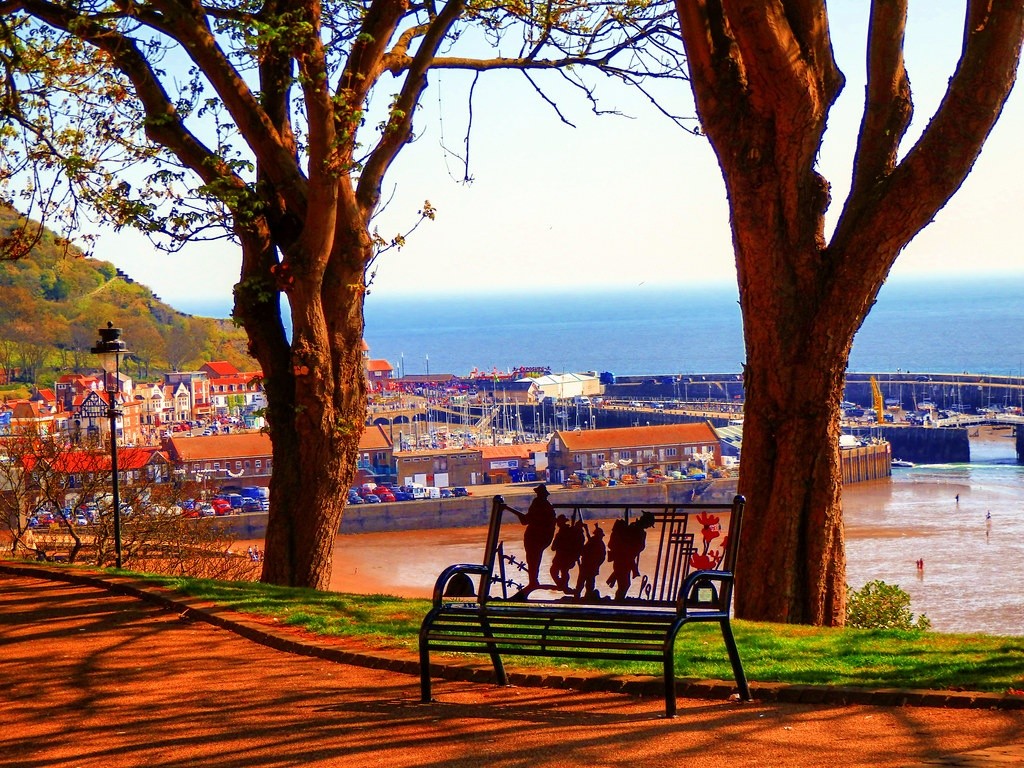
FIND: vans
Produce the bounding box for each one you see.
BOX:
[542,396,557,404]
[575,396,591,406]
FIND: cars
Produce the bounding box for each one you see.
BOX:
[26,483,468,527]
[591,398,604,406]
[127,416,239,447]
[629,401,640,407]
[651,403,663,409]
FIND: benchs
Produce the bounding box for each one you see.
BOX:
[417,485,752,718]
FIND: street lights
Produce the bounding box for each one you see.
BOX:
[90,320,136,570]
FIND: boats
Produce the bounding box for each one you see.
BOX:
[891,458,913,467]
[844,409,865,417]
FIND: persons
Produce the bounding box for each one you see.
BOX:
[919,558,923,569]
[955,493,960,501]
[916,560,919,568]
[985,510,991,519]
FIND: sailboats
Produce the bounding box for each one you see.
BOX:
[398,373,596,450]
[868,359,1024,437]
